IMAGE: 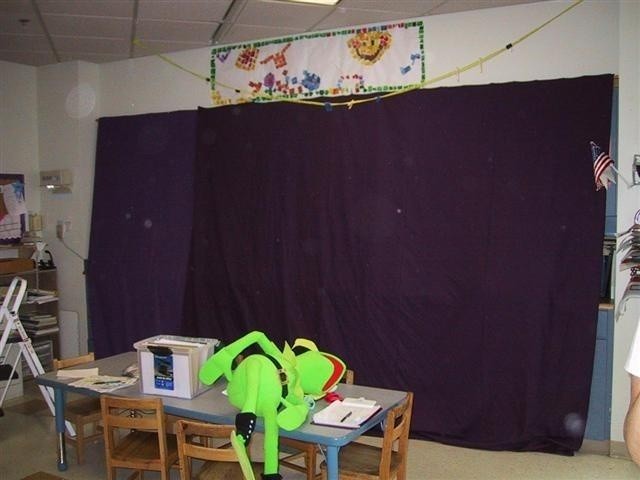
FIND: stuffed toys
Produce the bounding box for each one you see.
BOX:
[198,332,347,480]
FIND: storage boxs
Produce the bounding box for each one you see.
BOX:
[133,335,217,399]
[0,258,33,274]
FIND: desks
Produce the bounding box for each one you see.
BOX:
[37,351,407,480]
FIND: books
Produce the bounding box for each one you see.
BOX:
[0,285,59,337]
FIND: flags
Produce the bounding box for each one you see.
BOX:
[591,144,613,191]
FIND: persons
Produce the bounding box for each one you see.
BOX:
[623,320,640,467]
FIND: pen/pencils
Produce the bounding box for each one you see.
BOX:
[341,411,352,422]
[94,381,121,384]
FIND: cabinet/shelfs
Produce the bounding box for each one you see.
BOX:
[0,267,60,402]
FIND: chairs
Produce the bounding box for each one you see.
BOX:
[54,352,121,463]
[321,392,413,480]
[176,419,264,480]
[100,393,178,480]
[278,370,354,480]
